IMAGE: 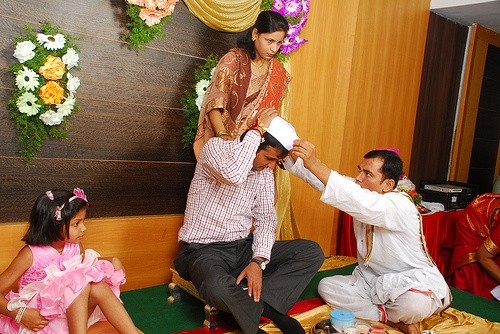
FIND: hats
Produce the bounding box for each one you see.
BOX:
[263,116,301,151]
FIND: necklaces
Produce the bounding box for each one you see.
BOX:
[252,59,265,71]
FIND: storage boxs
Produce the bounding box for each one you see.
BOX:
[423,184,477,209]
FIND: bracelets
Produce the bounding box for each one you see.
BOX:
[253,128,263,136]
[217,130,229,137]
[15,307,26,323]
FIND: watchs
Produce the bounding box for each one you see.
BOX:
[249,258,266,270]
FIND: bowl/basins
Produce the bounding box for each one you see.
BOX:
[344,321,386,334]
[329,309,356,332]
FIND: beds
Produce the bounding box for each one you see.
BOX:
[1,211,500,334]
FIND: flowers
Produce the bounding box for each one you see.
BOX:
[260,0,310,62]
[6,18,80,165]
[179,52,227,146]
[119,0,177,50]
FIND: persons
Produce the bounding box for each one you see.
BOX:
[447,193,500,299]
[174,107,325,334]
[281,139,453,334]
[193,11,290,162]
[0,189,138,334]
